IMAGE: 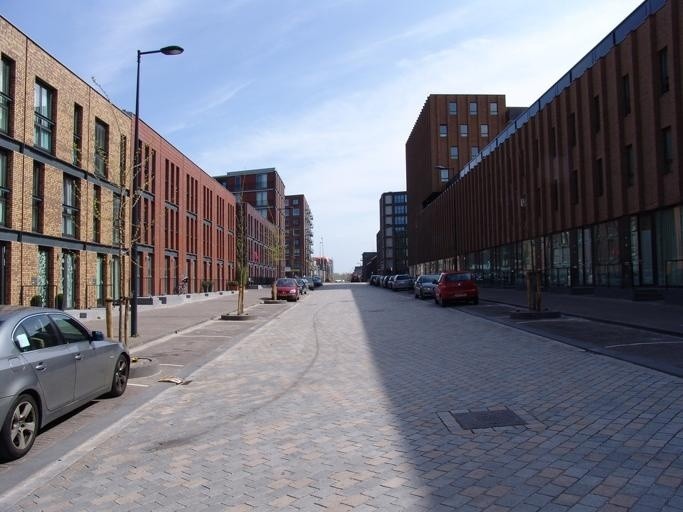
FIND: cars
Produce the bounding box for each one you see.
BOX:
[271,276,321,301]
[0,304,130,457]
[413,274,441,299]
[370,273,411,291]
[432,271,481,307]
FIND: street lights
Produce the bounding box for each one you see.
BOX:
[433,164,458,271]
[129,44,183,337]
